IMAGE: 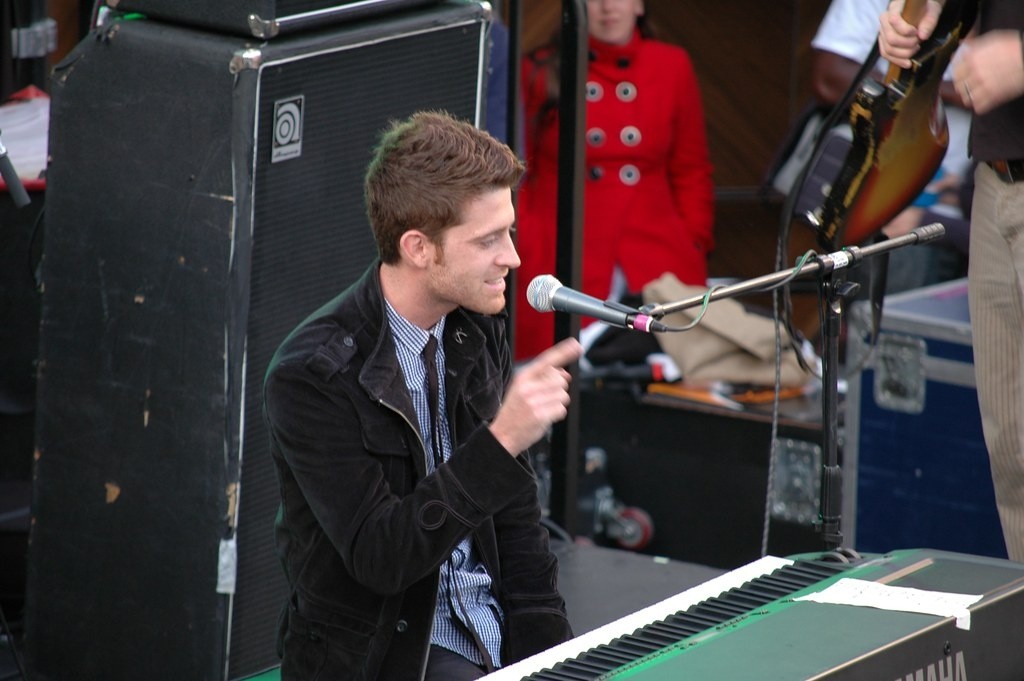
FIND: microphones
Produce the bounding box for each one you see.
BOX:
[526,274,667,333]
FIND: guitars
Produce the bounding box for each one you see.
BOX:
[816,1,982,253]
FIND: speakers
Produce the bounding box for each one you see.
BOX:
[15,16,486,681]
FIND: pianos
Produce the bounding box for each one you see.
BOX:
[469,544,1024,681]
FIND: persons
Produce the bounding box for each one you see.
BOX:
[258,108,576,681]
[511,0,718,372]
[803,0,1024,576]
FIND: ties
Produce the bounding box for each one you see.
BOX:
[421,334,441,469]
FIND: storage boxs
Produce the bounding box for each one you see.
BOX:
[842,279,1010,561]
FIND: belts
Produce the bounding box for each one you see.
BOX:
[987,157,1024,184]
